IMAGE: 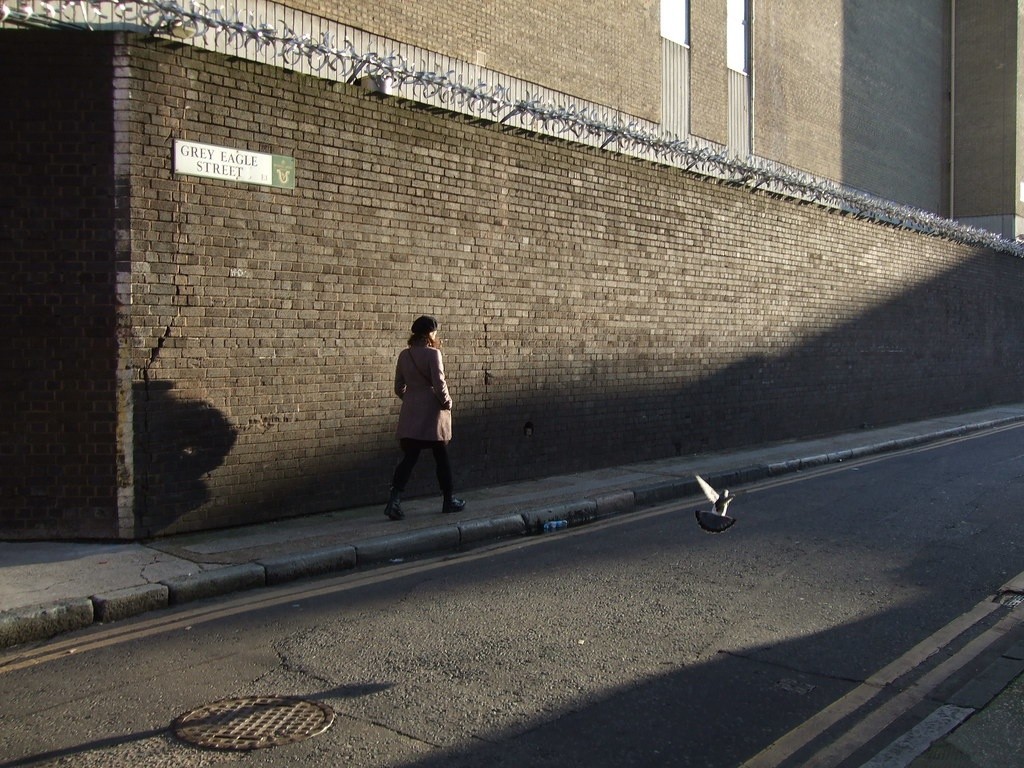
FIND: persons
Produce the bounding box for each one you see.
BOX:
[384,316,466,521]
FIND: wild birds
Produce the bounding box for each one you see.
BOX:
[696,475,736,533]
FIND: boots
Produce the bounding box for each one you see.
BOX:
[443,490,466,512]
[384,490,406,520]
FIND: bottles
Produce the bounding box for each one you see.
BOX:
[543,519,569,531]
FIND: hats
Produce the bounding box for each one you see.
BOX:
[412,315,438,332]
